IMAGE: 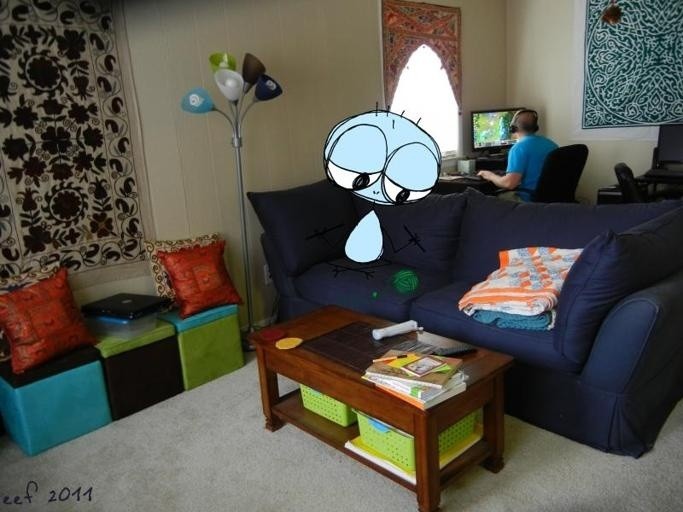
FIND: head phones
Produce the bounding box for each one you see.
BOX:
[508,110,539,133]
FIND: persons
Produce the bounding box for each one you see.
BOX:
[476,110,560,204]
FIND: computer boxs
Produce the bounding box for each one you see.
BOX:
[476,157,507,170]
[598,183,647,204]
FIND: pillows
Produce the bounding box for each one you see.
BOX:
[0,261,63,364]
[450,187,683,290]
[1,267,97,376]
[247,177,358,276]
[158,241,244,317]
[552,206,682,368]
[354,193,467,281]
[145,233,222,314]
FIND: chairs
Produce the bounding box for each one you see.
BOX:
[490,144,589,202]
[613,162,646,204]
[652,123,683,169]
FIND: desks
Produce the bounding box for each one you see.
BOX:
[436,172,510,196]
[635,169,683,201]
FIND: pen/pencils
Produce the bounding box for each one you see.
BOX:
[372,355,408,363]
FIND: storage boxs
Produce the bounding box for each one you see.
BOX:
[84,314,157,339]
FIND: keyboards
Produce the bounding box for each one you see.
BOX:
[464,170,505,181]
[645,171,683,177]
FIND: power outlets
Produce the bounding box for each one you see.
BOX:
[263,264,274,287]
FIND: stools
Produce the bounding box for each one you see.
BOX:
[89,319,185,422]
[0,346,113,457]
[164,306,245,392]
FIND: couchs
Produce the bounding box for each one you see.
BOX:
[259,230,683,459]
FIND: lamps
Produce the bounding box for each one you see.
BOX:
[180,50,283,353]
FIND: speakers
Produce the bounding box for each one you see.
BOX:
[652,147,663,169]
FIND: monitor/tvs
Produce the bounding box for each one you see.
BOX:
[472,108,525,156]
[658,123,683,164]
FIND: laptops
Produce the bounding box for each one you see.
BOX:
[81,293,171,320]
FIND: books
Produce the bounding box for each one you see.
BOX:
[360,348,467,410]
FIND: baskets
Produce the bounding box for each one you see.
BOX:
[349,407,474,472]
[300,382,357,427]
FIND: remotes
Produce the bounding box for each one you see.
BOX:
[434,344,476,355]
